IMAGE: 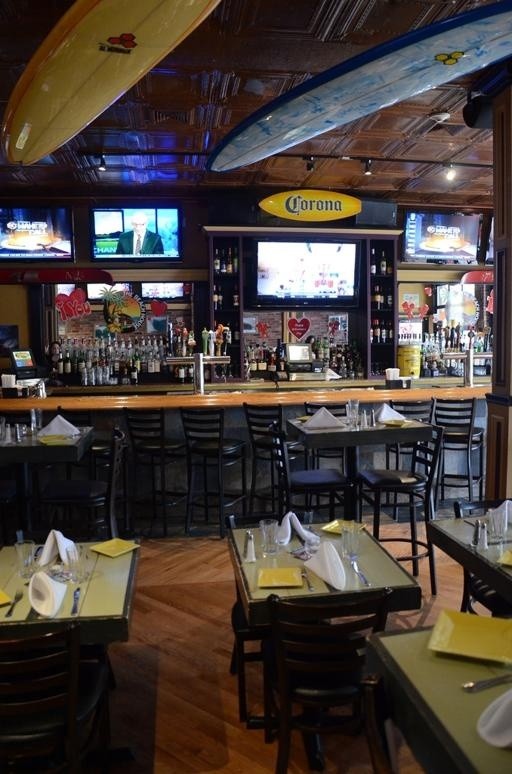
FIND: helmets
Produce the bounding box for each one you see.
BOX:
[37,434,72,446]
[258,566,303,588]
[320,519,366,535]
[90,538,141,558]
[382,419,412,428]
[1,589,11,606]
[429,607,511,664]
[296,416,312,422]
[496,550,512,565]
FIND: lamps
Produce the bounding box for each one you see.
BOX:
[83,150,493,182]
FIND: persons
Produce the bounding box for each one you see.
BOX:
[49,342,61,355]
[114,213,164,254]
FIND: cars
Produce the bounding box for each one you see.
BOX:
[15,540,35,579]
[20,424,26,434]
[340,524,360,558]
[347,404,358,426]
[31,407,36,430]
[348,400,360,425]
[65,546,89,583]
[22,388,29,397]
[259,519,279,556]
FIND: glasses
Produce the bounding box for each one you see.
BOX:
[83,150,493,182]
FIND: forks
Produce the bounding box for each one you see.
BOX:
[4,590,24,618]
[300,567,314,593]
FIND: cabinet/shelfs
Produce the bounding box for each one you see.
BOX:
[388,287,393,308]
[387,323,394,341]
[221,249,226,271]
[479,522,488,550]
[373,285,381,310]
[257,351,267,370]
[175,336,182,356]
[247,344,255,362]
[173,365,194,385]
[380,320,386,343]
[471,519,481,546]
[275,339,284,361]
[356,361,364,379]
[330,355,337,371]
[246,535,255,561]
[330,338,336,354]
[268,352,276,372]
[216,284,223,308]
[422,319,458,353]
[387,259,393,274]
[182,340,187,356]
[371,363,375,376]
[431,361,439,377]
[37,409,43,429]
[315,336,325,361]
[233,247,239,271]
[226,246,233,273]
[361,410,368,428]
[242,531,250,557]
[205,366,211,384]
[337,345,343,361]
[380,286,385,308]
[370,248,376,274]
[420,361,431,377]
[369,409,376,427]
[262,342,268,350]
[249,349,257,371]
[53,335,168,387]
[349,361,355,379]
[351,340,357,354]
[379,250,386,273]
[486,359,491,376]
[213,284,219,310]
[214,247,220,272]
[5,424,12,444]
[232,285,239,306]
[373,319,381,343]
[473,341,478,352]
[323,337,330,359]
[478,337,485,352]
[254,344,262,353]
[339,357,348,378]
[370,322,374,343]
[376,364,380,375]
[14,424,21,442]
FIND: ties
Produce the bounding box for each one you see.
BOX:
[398,209,495,263]
[88,206,188,263]
[245,239,362,312]
[0,201,77,263]
[7,347,37,371]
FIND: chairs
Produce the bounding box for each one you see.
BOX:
[122,403,190,538]
[0,398,136,544]
[435,399,484,513]
[267,428,354,524]
[386,397,436,523]
[177,406,249,540]
[242,402,309,528]
[304,398,353,512]
[266,588,394,773]
[0,621,116,773]
[453,499,486,593]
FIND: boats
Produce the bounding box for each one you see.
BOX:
[461,93,493,131]
[356,197,398,227]
[208,204,262,225]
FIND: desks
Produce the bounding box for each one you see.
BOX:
[287,410,433,516]
[368,619,511,773]
[228,522,422,745]
[429,517,510,619]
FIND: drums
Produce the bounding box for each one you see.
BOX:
[351,561,370,586]
[70,587,80,615]
